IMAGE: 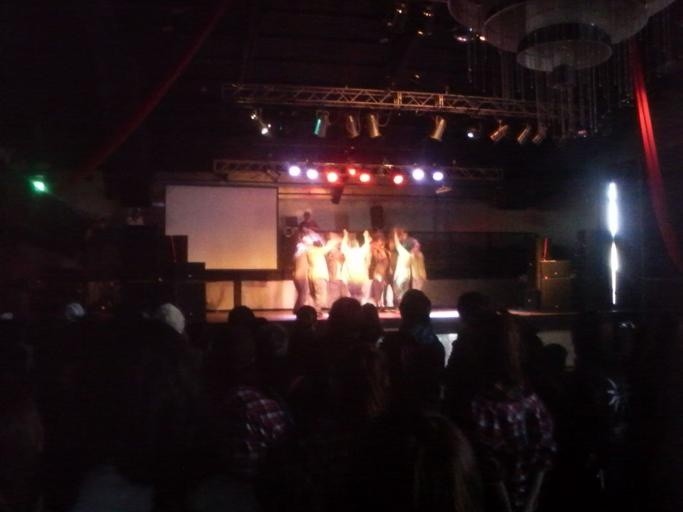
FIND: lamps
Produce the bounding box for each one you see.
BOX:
[486,113,548,147]
[426,110,447,142]
[310,105,383,141]
